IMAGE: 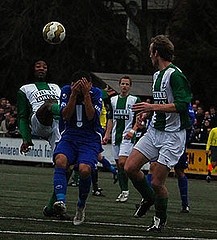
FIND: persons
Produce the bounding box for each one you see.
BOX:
[16,60,66,215]
[205,127,217,182]
[0,95,217,143]
[52,69,103,226]
[102,75,144,204]
[124,35,194,233]
[97,96,119,184]
[146,100,194,213]
[68,150,119,198]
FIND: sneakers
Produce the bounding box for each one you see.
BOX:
[90,187,102,195]
[52,201,67,220]
[146,216,168,232]
[134,194,155,217]
[115,190,129,203]
[180,207,190,213]
[73,203,86,226]
[42,206,60,219]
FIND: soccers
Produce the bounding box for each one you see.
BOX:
[42,21,66,46]
